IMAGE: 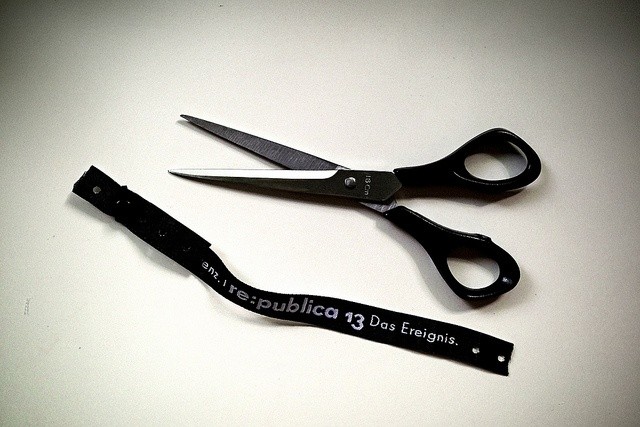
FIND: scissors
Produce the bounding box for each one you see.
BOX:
[168,114,542,307]
